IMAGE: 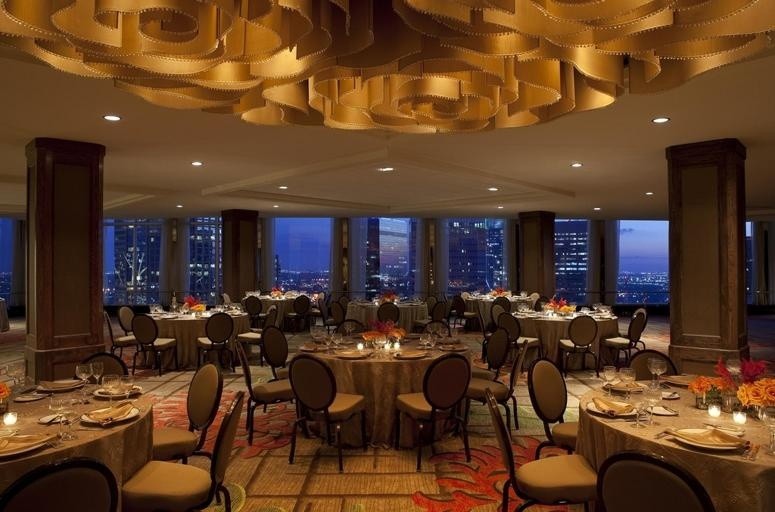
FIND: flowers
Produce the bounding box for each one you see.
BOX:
[182,295,199,306]
[543,296,567,309]
[190,304,206,311]
[369,319,394,337]
[360,331,386,341]
[739,379,774,409]
[270,287,282,297]
[558,305,575,314]
[388,328,405,341]
[715,359,767,387]
[491,287,507,296]
[0,380,10,398]
[689,375,727,393]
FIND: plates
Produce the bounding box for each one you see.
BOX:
[587,402,637,416]
[647,407,679,416]
[603,381,646,393]
[299,344,470,358]
[661,392,680,400]
[192,311,213,318]
[706,424,744,436]
[0,379,143,458]
[665,375,691,386]
[160,314,178,319]
[674,429,740,450]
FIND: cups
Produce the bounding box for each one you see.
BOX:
[707,404,722,419]
[731,411,746,425]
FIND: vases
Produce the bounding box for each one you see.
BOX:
[0,397,9,414]
[757,406,775,421]
[696,391,711,409]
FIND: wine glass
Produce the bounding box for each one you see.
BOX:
[643,386,662,426]
[7,362,138,441]
[216,303,241,314]
[631,396,646,429]
[331,322,459,360]
[758,402,775,456]
[604,358,667,402]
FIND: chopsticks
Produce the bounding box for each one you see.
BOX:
[667,392,677,397]
[662,405,676,413]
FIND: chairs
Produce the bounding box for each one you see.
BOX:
[311,294,332,326]
[121,390,245,511]
[597,453,715,511]
[73,353,128,381]
[486,388,599,512]
[528,358,580,461]
[490,302,506,329]
[286,295,310,333]
[533,296,550,310]
[197,312,235,373]
[452,339,529,438]
[625,349,677,379]
[335,319,365,334]
[310,291,326,327]
[597,312,645,372]
[318,298,339,334]
[492,296,511,313]
[251,303,278,333]
[261,325,292,380]
[330,301,345,324]
[377,302,400,323]
[236,309,278,367]
[531,293,540,306]
[1,457,119,512]
[413,301,446,333]
[617,308,648,363]
[428,298,454,337]
[419,321,451,337]
[393,353,473,472]
[235,341,309,446]
[337,296,350,313]
[424,295,438,314]
[289,353,368,473]
[453,295,477,333]
[119,306,135,335]
[245,296,266,328]
[131,314,178,377]
[469,326,511,406]
[152,362,223,465]
[498,312,541,373]
[560,315,600,378]
[104,310,147,370]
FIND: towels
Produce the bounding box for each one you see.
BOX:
[604,380,642,389]
[98,386,140,395]
[39,381,84,390]
[665,426,745,450]
[661,373,699,385]
[592,396,635,418]
[329,351,370,357]
[396,350,431,358]
[0,433,59,454]
[84,401,133,426]
